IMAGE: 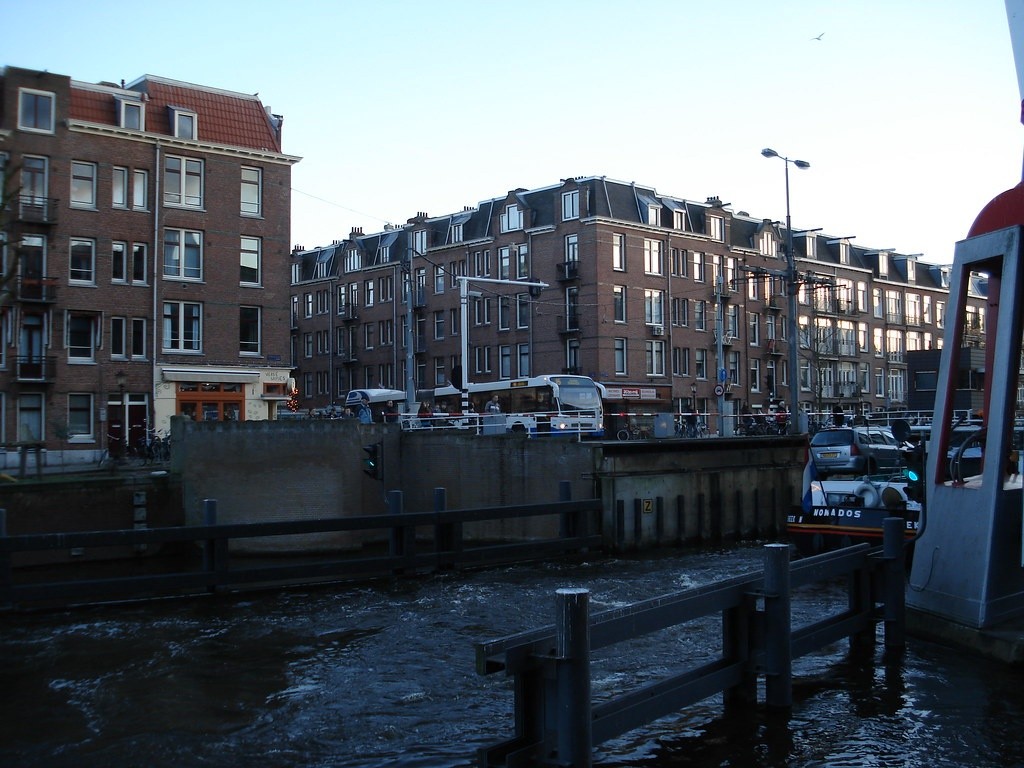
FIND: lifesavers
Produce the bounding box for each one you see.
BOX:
[855,483,881,508]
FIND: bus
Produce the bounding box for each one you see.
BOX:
[344,372,608,439]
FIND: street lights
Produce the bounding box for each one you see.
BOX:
[690,382,698,413]
[759,147,813,433]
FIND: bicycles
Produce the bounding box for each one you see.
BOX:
[133,427,172,467]
[615,415,711,442]
[735,414,833,436]
[97,434,139,470]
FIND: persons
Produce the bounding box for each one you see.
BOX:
[417,400,474,427]
[306,398,372,424]
[832,402,844,428]
[686,404,699,439]
[776,402,788,434]
[485,395,501,413]
[382,400,397,424]
[741,402,752,437]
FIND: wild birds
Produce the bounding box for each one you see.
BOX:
[812,32,824,40]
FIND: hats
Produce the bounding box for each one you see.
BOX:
[361,399,369,407]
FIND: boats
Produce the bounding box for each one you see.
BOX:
[784,415,1024,542]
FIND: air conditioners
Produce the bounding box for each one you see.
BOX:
[652,326,665,336]
